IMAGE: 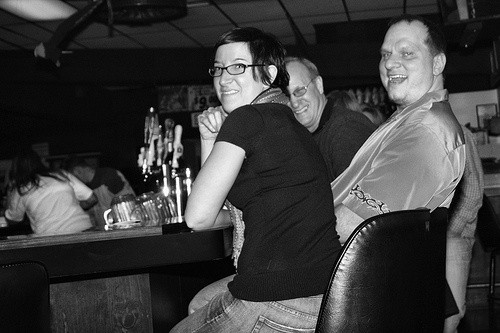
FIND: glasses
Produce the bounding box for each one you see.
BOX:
[208,63,266,78]
[290,77,315,98]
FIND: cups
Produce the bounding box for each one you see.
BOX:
[104,192,177,226]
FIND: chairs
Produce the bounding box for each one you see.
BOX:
[315,206,448,333]
[473,194,500,294]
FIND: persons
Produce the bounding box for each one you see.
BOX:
[4,144,97,237]
[280,52,375,175]
[144,25,340,333]
[323,11,483,333]
[70,158,136,229]
